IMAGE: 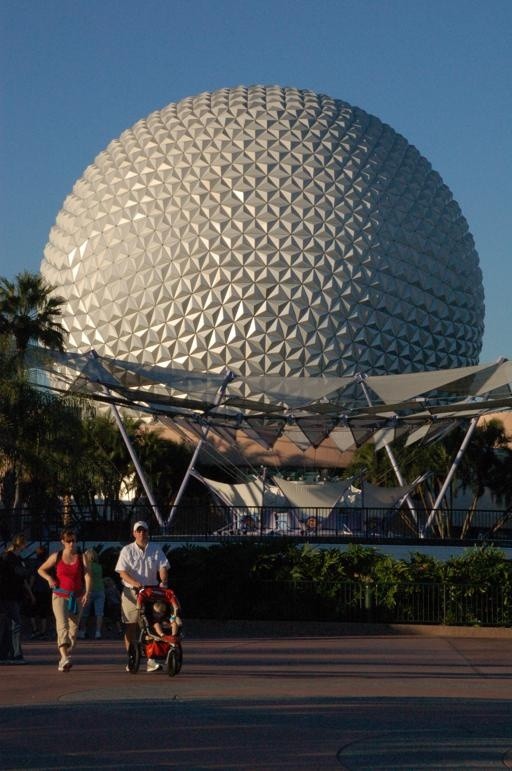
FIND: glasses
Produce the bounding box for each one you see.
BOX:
[65,539,74,542]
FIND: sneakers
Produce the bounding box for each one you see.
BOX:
[95,631,101,638]
[78,631,85,638]
[147,658,163,673]
[58,656,72,673]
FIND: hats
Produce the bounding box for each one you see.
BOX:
[134,520,148,530]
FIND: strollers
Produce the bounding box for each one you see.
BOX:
[127,585,183,677]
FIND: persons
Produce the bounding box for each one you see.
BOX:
[114,520,170,673]
[78,548,107,641]
[0,532,49,666]
[149,599,179,637]
[37,528,93,673]
